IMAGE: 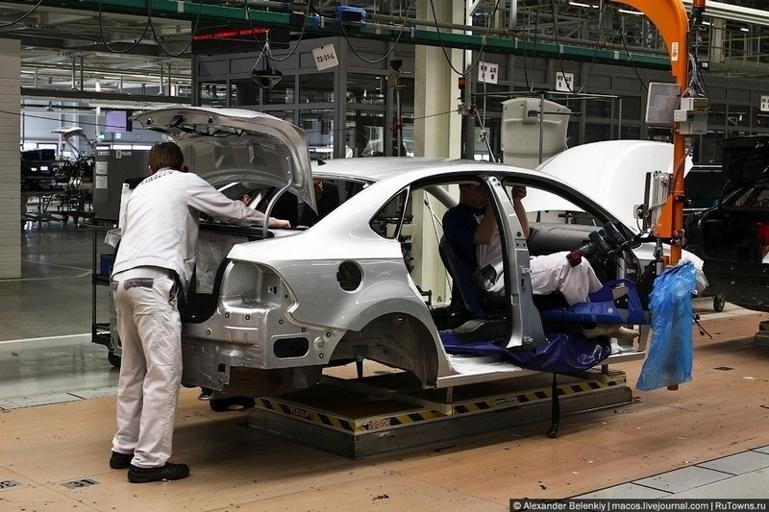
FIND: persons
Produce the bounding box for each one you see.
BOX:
[441,182,640,341]
[110,138,292,484]
[314,180,339,219]
[242,189,268,213]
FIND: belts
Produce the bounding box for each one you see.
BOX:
[110,278,176,296]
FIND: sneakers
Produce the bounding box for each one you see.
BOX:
[109,450,135,469]
[583,322,640,342]
[127,464,190,485]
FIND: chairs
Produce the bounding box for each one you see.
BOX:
[437,233,570,317]
